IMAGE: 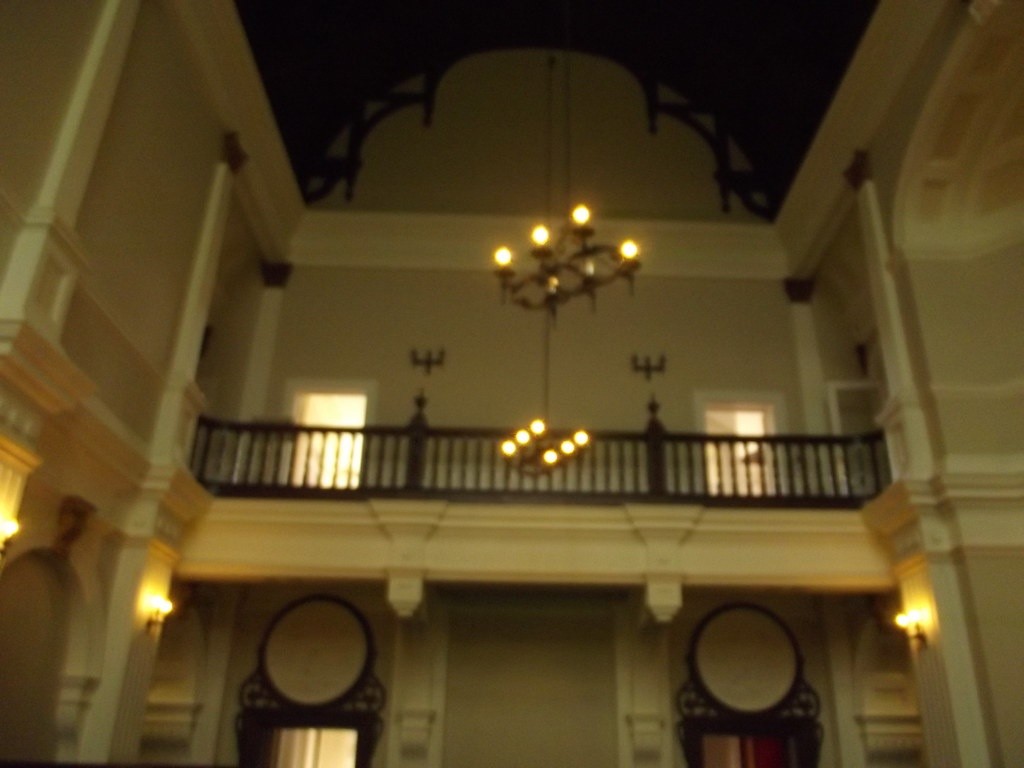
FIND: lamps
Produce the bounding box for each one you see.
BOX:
[494,56,644,321]
[893,607,929,644]
[146,593,173,629]
[495,57,595,469]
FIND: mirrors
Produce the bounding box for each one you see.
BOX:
[264,597,369,707]
[696,610,799,712]
